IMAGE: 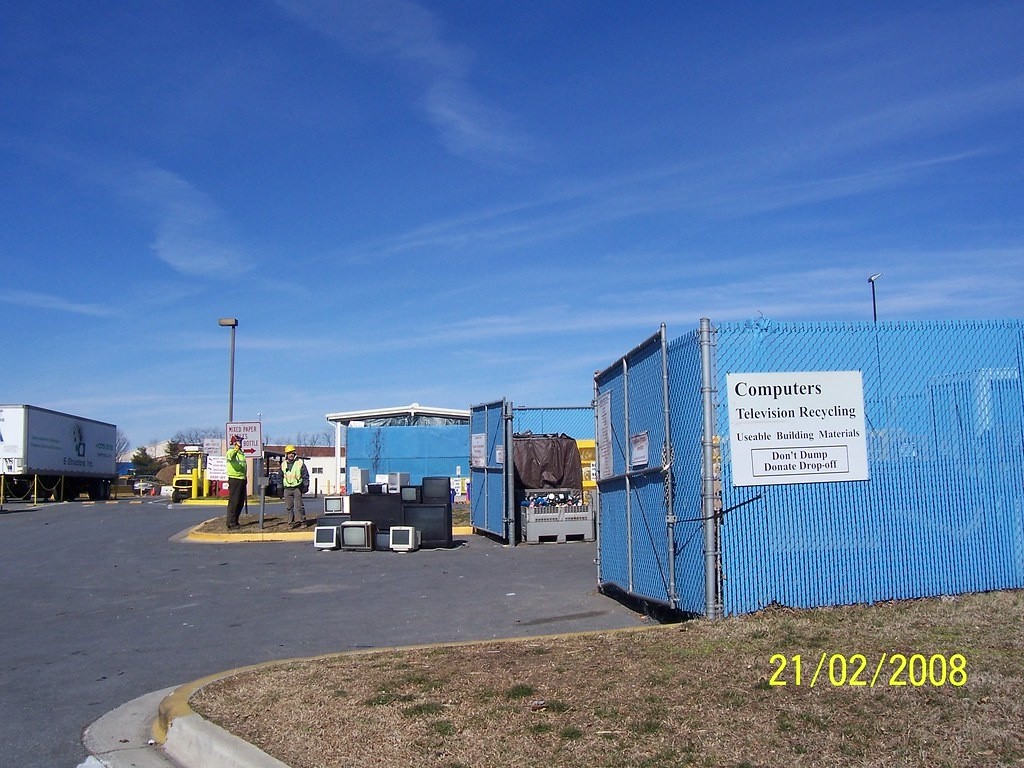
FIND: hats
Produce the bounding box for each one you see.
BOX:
[231,436,244,443]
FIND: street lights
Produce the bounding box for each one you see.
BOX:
[218,317,238,421]
[867,272,886,399]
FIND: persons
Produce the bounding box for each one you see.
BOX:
[278,445,310,529]
[224,435,248,530]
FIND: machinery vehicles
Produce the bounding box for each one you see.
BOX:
[170,451,211,503]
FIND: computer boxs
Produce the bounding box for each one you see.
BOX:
[388,472,410,493]
[353,469,369,493]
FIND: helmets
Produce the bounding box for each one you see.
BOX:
[285,445,296,453]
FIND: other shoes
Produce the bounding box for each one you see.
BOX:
[301,522,307,528]
[226,523,244,531]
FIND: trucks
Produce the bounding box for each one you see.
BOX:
[1,403,117,503]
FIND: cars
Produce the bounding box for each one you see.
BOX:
[265,472,279,496]
[119,476,131,479]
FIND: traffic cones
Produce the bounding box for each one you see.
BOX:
[151,486,156,495]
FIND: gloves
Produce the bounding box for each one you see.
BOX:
[303,483,309,491]
[277,483,282,489]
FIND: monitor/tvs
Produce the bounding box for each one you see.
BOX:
[314,477,452,554]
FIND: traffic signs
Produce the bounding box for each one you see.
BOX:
[206,456,229,481]
[226,421,262,459]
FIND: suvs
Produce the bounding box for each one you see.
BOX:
[133,474,166,496]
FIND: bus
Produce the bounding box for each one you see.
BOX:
[127,469,136,476]
